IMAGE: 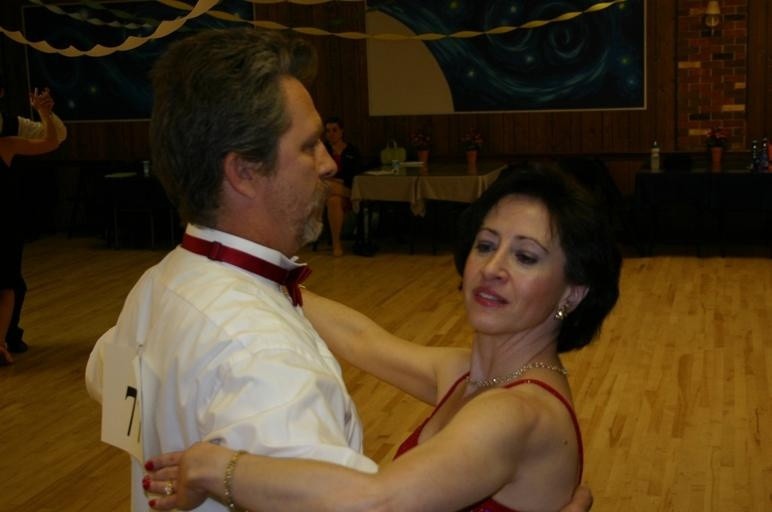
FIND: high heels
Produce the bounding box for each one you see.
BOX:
[0,344,14,365]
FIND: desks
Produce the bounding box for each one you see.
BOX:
[690,154,770,256]
[633,159,683,255]
[102,171,176,251]
[354,162,505,255]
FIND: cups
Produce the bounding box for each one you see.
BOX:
[391,160,400,173]
[139,160,153,181]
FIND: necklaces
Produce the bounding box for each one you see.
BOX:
[463,360,572,390]
[162,479,175,497]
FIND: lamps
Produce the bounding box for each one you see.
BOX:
[705,0,722,29]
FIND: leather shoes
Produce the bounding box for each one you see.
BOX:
[6,334,28,353]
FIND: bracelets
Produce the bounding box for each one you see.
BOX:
[298,283,307,290]
[222,449,251,512]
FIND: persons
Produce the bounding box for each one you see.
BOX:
[0,86,60,367]
[142,154,621,512]
[84,22,593,512]
[321,116,370,259]
[0,84,68,355]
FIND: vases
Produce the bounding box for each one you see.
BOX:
[420,151,429,160]
[465,150,477,163]
[710,146,724,173]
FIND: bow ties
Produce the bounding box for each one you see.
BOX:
[179,234,312,307]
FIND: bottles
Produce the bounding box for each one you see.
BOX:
[650,140,661,172]
[749,138,769,169]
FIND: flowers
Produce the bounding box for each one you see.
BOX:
[413,134,431,151]
[704,126,731,153]
[458,126,484,150]
